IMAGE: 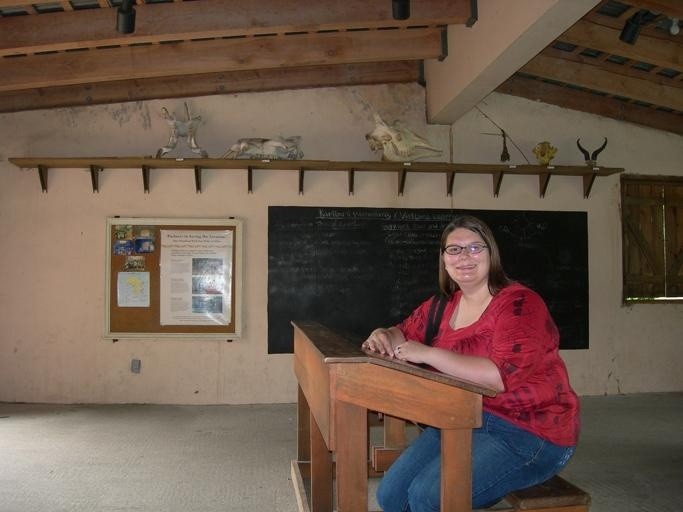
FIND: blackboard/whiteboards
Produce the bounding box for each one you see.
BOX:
[268,206,589,355]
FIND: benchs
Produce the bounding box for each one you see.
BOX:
[411,421,591,512]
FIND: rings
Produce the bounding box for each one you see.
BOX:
[397,347,402,353]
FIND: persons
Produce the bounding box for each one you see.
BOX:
[359,211,580,512]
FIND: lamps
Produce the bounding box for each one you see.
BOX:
[115,0,136,34]
[670,18,680,35]
[391,0,410,20]
[620,8,647,46]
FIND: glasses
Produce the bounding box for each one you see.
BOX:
[439,241,491,257]
